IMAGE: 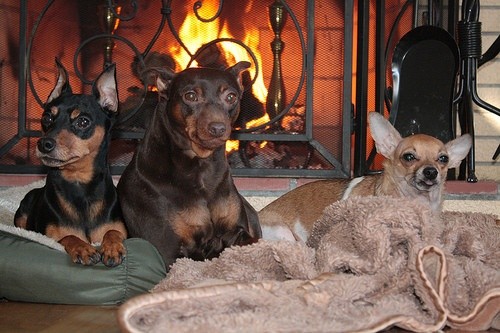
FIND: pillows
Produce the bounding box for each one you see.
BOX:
[1,230,169,306]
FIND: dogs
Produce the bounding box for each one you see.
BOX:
[117,60,262,261]
[258,111,472,243]
[13,59,128,267]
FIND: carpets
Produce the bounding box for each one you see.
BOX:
[0,176,122,253]
[117,194,500,333]
